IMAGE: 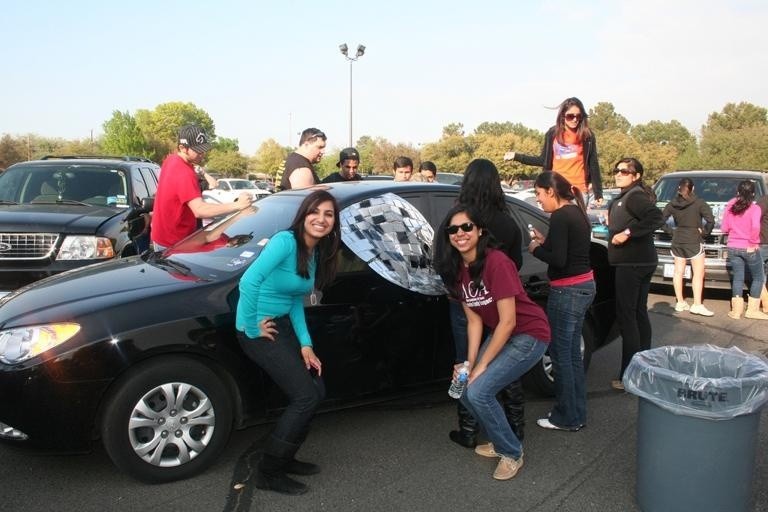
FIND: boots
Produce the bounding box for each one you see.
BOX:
[760,287,768,313]
[745,296,768,320]
[727,296,745,320]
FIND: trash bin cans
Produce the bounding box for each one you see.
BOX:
[623,345,768,512]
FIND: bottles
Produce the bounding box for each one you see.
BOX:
[596,212,607,230]
[447,361,469,400]
[527,223,538,242]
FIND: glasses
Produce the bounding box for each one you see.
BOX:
[445,222,476,233]
[560,113,584,122]
[613,167,634,176]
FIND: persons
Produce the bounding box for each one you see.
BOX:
[661,177,715,316]
[280,128,327,190]
[434,205,551,480]
[721,181,768,320]
[529,172,597,431]
[322,148,363,183]
[150,126,253,253]
[755,195,768,313]
[419,161,439,183]
[236,190,340,495]
[434,160,524,448]
[504,97,603,210]
[192,163,219,230]
[393,157,413,182]
[601,158,665,390]
[164,205,258,258]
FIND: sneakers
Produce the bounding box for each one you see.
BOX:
[690,305,713,317]
[674,301,690,312]
[491,456,526,481]
[611,379,627,391]
[512,428,525,439]
[278,458,320,475]
[536,411,586,430]
[474,442,499,457]
[258,473,308,496]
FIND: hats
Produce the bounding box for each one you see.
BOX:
[179,126,213,155]
[336,147,361,167]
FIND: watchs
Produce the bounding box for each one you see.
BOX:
[625,228,630,237]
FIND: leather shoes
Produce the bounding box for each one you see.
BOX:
[449,429,477,447]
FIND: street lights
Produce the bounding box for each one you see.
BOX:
[339,43,367,146]
